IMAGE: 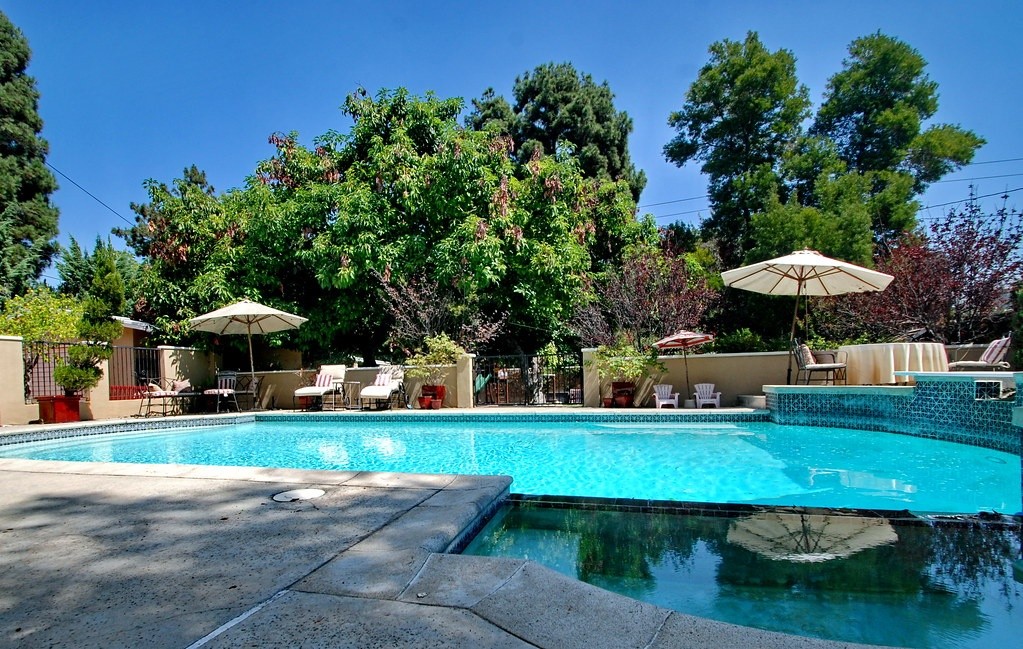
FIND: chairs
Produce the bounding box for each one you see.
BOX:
[216,372,240,414]
[652,384,680,408]
[950,337,1011,370]
[693,383,721,408]
[292,365,350,411]
[793,344,847,385]
[360,364,410,411]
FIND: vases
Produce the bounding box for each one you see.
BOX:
[430,399,442,410]
[417,396,432,409]
[603,398,615,407]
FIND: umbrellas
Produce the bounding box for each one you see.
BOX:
[652,330,714,399]
[189,296,309,408]
[726,512,899,563]
[721,247,895,385]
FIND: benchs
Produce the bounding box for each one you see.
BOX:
[137,377,204,418]
[214,376,265,411]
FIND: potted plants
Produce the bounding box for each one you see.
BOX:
[403,331,469,407]
[34,294,125,424]
[584,336,670,407]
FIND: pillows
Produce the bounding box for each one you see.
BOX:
[375,373,392,386]
[248,377,259,390]
[148,383,163,391]
[314,373,333,387]
[172,378,190,391]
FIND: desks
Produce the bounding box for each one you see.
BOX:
[835,343,948,386]
[332,381,361,411]
